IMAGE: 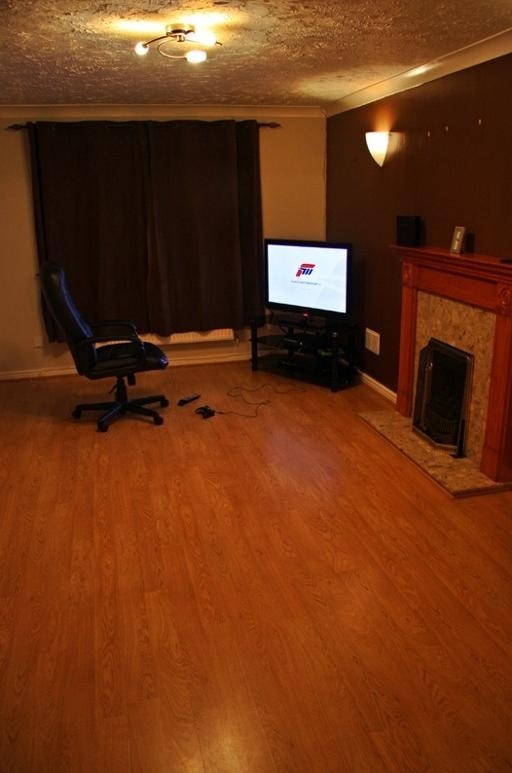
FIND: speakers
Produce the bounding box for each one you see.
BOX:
[397,215,422,246]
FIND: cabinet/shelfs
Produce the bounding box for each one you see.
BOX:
[251,316,354,390]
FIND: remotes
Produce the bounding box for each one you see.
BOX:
[177,394,200,407]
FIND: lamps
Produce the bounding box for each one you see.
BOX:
[134,25,223,65]
[364,131,391,167]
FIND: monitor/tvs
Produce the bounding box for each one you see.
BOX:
[264,239,352,328]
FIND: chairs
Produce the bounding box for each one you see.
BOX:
[33,250,169,430]
